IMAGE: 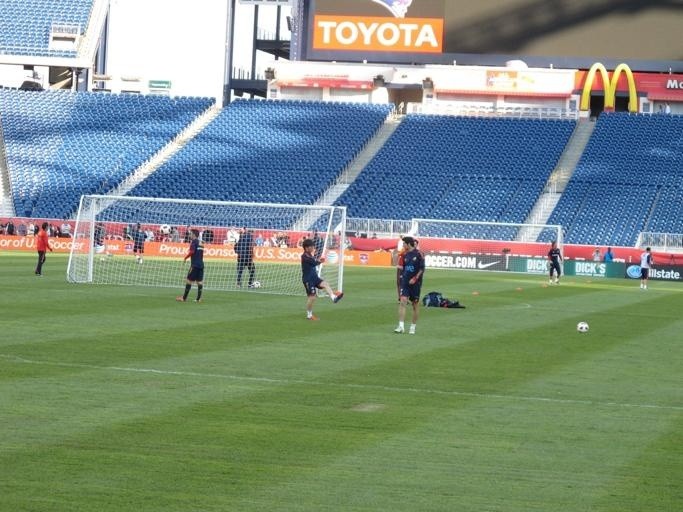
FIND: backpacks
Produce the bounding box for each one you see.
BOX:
[423,292,442,306]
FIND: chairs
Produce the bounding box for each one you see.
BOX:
[1,1,110,83]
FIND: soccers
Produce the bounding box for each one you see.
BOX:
[253,282,260,289]
[160,225,170,235]
[577,322,589,332]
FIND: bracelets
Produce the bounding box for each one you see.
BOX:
[412,276,418,282]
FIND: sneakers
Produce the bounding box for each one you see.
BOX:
[176,296,184,301]
[394,326,405,333]
[409,326,416,334]
[307,316,320,320]
[333,293,343,303]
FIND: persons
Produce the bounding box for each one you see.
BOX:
[233,227,256,289]
[174,228,204,302]
[300,239,344,320]
[421,291,466,308]
[639,247,653,290]
[33,222,53,276]
[658,101,672,114]
[603,246,614,261]
[397,100,404,115]
[591,246,600,262]
[547,241,563,285]
[393,236,424,335]
[1,216,423,265]
